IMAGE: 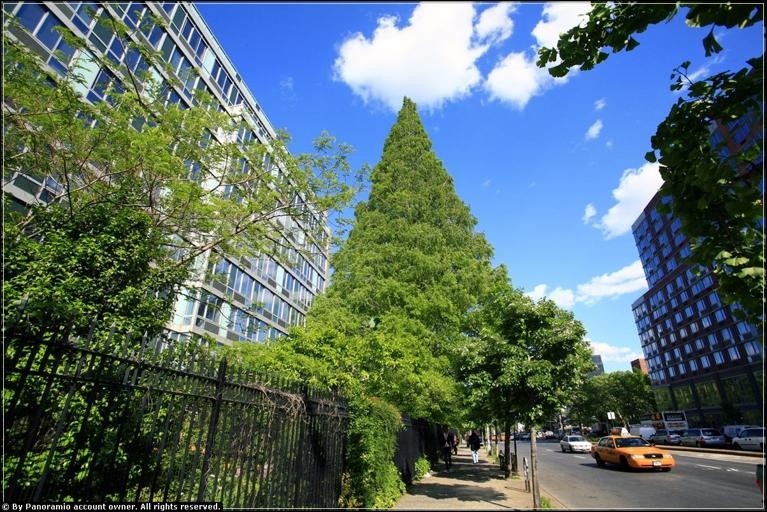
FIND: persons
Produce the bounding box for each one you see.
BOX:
[440,424,453,474]
[453,434,459,455]
[467,429,480,463]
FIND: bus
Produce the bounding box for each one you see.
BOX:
[637,410,689,431]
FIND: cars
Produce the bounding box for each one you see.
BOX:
[653,427,766,452]
[560,434,592,454]
[591,435,676,473]
[492,432,529,441]
